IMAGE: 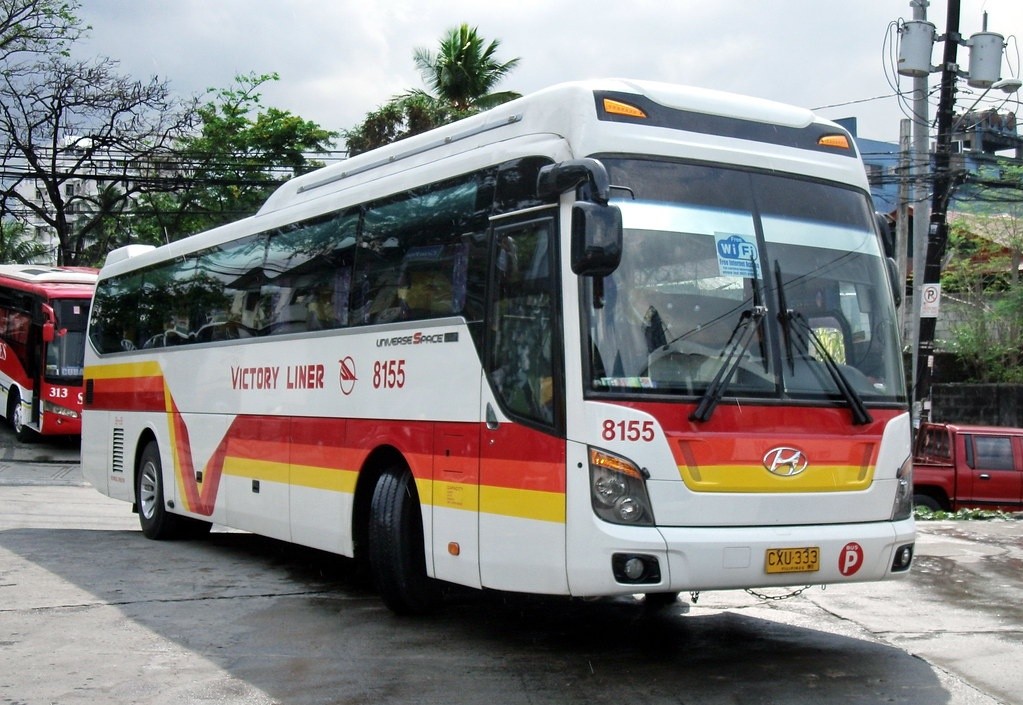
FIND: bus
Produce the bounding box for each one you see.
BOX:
[80,74,915,619]
[0,263,103,444]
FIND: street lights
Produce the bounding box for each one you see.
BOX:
[913,78,1022,423]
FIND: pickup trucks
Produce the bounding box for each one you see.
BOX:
[910,422,1023,514]
